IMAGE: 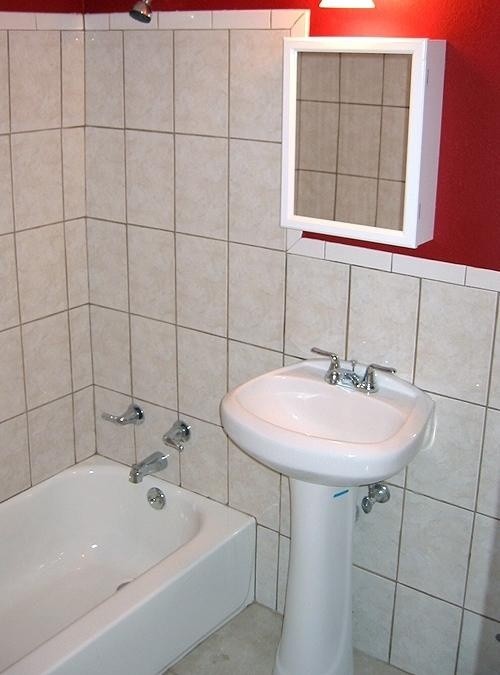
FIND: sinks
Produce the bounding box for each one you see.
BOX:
[220,364,435,487]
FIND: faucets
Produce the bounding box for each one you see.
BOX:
[129,451,167,483]
[329,366,360,386]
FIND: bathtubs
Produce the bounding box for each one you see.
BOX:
[0,454,256,675]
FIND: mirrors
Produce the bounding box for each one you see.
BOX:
[279,33,447,248]
